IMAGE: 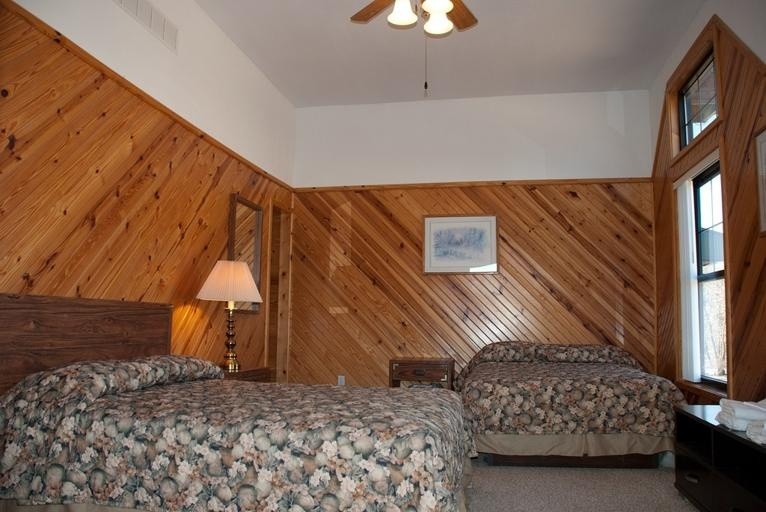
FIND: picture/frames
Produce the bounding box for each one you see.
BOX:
[752,125,766,240]
[422,214,500,275]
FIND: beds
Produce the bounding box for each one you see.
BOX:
[0,291,468,511]
[453,340,677,470]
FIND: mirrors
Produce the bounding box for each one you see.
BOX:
[224,192,265,315]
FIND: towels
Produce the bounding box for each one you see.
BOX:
[714,397,766,445]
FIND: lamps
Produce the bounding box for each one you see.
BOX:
[195,259,264,369]
[349,0,480,40]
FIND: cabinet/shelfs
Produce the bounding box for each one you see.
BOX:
[672,405,766,512]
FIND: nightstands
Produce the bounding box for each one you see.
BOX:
[219,363,272,383]
[389,358,457,391]
